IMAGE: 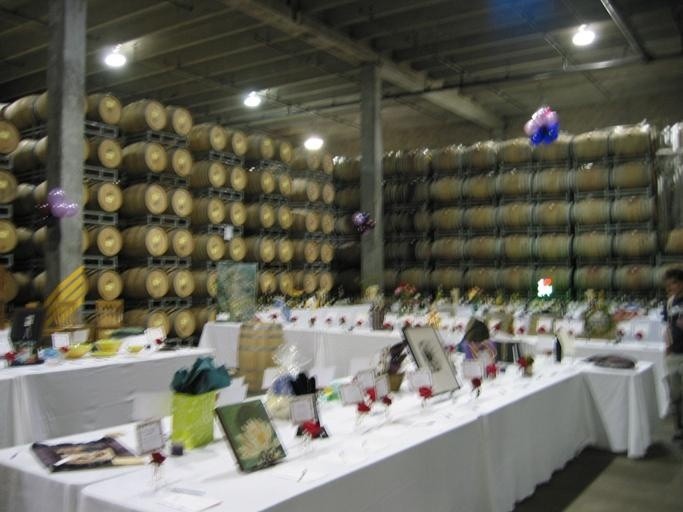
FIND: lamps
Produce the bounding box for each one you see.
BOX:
[106,45,127,69]
[246,90,263,111]
[572,23,596,48]
[306,132,325,151]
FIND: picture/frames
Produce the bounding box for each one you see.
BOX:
[406,325,462,405]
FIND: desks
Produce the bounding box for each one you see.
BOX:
[0,348,221,449]
[35,300,671,492]
[1,349,599,512]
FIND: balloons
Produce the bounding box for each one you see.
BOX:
[64,199,80,220]
[45,188,67,205]
[524,107,559,144]
[47,203,69,217]
[353,212,365,228]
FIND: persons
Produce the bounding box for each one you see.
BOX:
[661,267,683,443]
